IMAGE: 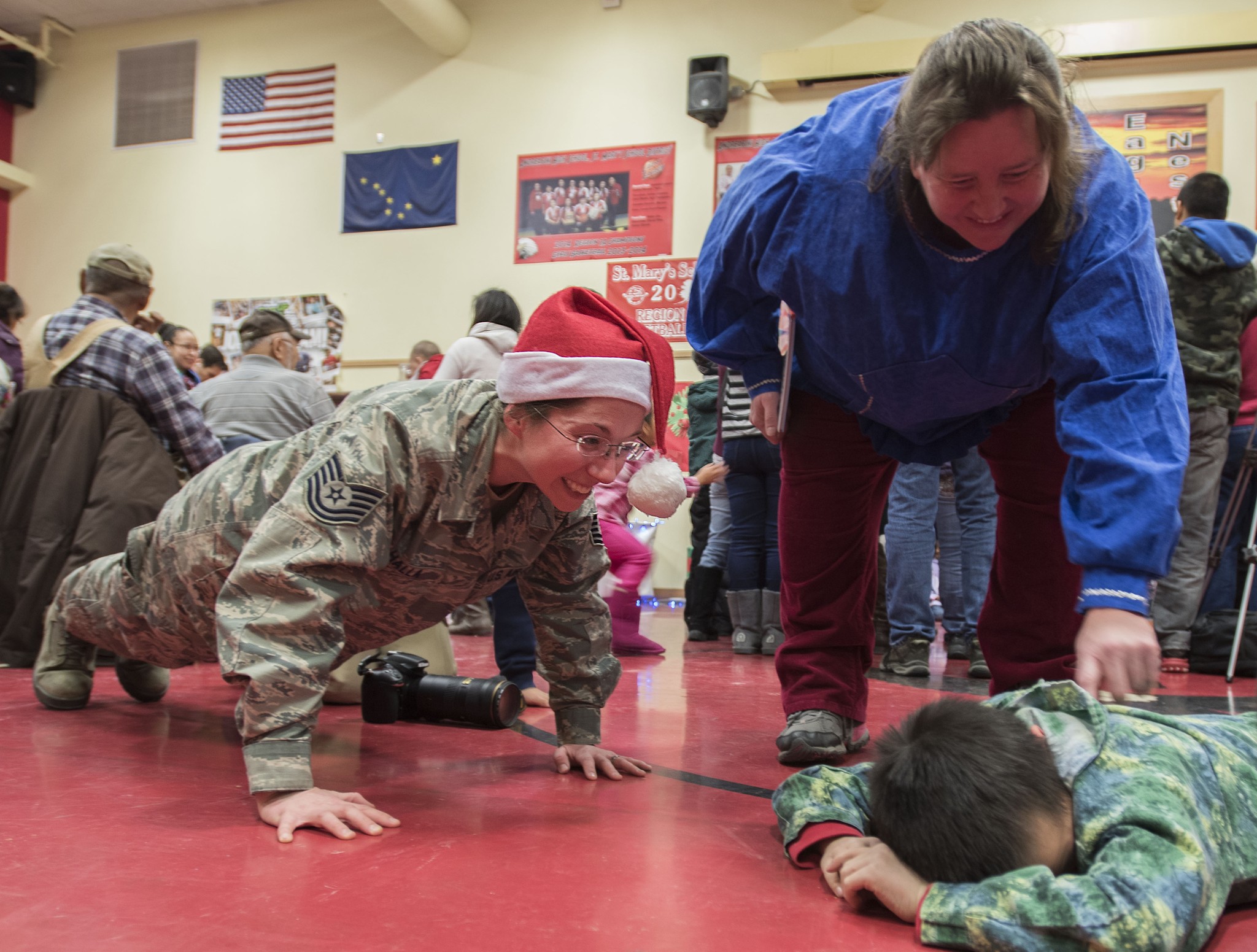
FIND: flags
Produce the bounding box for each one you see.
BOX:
[218,62,335,152]
[338,138,456,235]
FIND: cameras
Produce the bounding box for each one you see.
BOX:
[357,650,523,729]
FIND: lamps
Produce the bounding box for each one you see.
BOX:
[687,55,728,127]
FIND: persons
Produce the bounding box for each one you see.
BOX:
[685,18,1190,770]
[25,241,336,486]
[682,173,1257,679]
[432,289,522,637]
[586,411,729,658]
[0,281,28,409]
[774,680,1257,952]
[398,340,446,381]
[30,286,685,843]
[527,176,621,234]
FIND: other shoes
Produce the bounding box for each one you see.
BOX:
[928,600,944,622]
[448,598,493,636]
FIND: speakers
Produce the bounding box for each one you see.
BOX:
[0,44,37,106]
[685,54,730,125]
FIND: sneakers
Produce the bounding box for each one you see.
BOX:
[875,633,889,654]
[879,636,930,676]
[968,634,991,678]
[1161,649,1190,672]
[115,657,170,703]
[944,632,971,660]
[775,709,869,766]
[32,596,99,709]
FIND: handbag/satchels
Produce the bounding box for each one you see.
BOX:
[1187,608,1257,678]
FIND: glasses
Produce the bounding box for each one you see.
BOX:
[171,342,199,353]
[523,403,651,461]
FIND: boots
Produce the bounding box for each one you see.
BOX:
[604,595,666,655]
[725,588,764,653]
[685,565,721,641]
[762,591,784,655]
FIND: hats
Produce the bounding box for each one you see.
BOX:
[87,243,153,285]
[495,286,687,519]
[240,309,312,340]
